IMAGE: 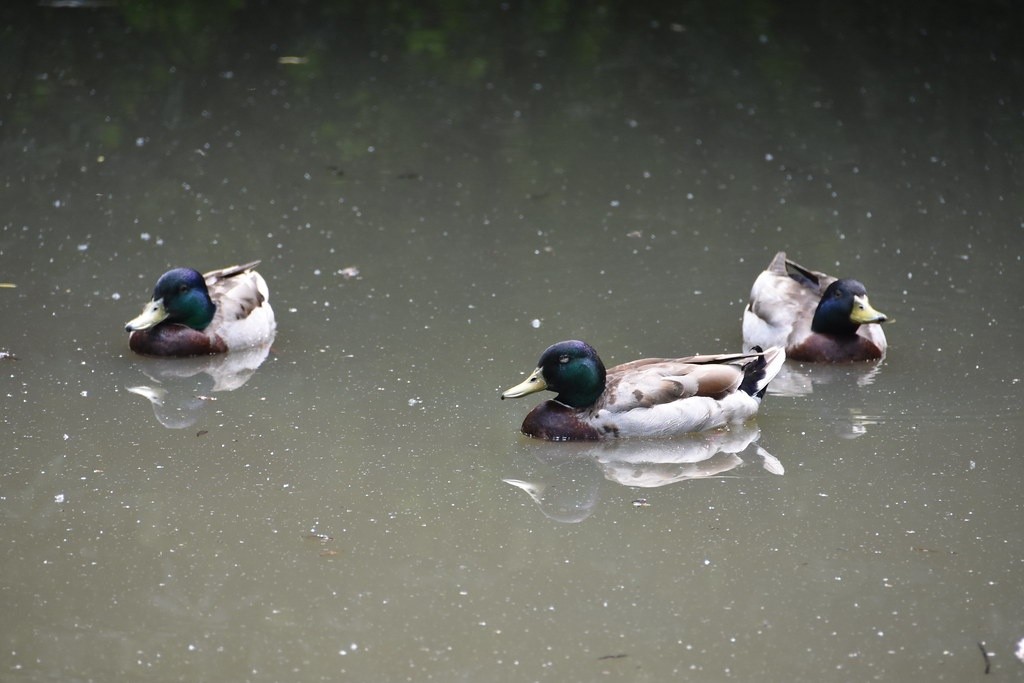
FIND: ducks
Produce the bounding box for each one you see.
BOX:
[124,261,277,359]
[741,250,891,363]
[500,339,786,444]
